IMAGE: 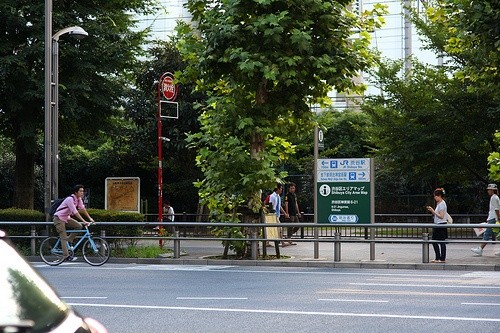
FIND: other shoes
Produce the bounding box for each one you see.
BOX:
[153,227,159,229]
[430,259,446,263]
[265,244,273,247]
[281,241,297,247]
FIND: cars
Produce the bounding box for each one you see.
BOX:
[0,229,107,333]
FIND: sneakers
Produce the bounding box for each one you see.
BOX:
[64,255,70,260]
[494,251,500,256]
[470,247,483,255]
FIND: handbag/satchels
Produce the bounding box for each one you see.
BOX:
[447,212,454,224]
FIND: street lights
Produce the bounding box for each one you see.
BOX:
[52,25,89,200]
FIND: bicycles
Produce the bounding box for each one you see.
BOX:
[39,216,112,267]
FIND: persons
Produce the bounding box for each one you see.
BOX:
[53,185,94,262]
[426,188,453,263]
[153,201,175,240]
[470,184,500,257]
[263,182,301,247]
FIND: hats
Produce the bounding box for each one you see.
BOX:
[486,183,498,189]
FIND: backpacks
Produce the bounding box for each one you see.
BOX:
[263,194,270,207]
[48,196,79,217]
